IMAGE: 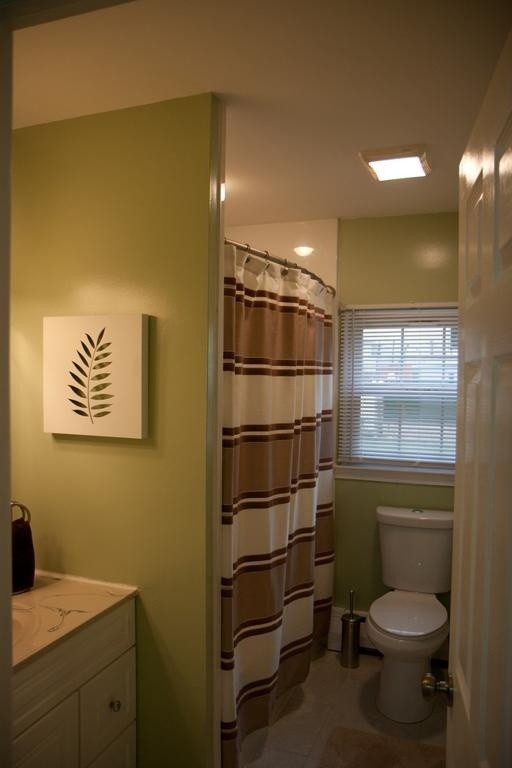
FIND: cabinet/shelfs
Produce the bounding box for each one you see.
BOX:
[9,596,141,768]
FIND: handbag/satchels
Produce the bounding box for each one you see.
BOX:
[11,500,35,596]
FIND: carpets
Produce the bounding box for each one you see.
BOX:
[314,723,447,768]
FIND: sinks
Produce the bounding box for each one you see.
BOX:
[11,603,40,651]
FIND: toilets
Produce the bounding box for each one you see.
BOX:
[365,505,453,723]
